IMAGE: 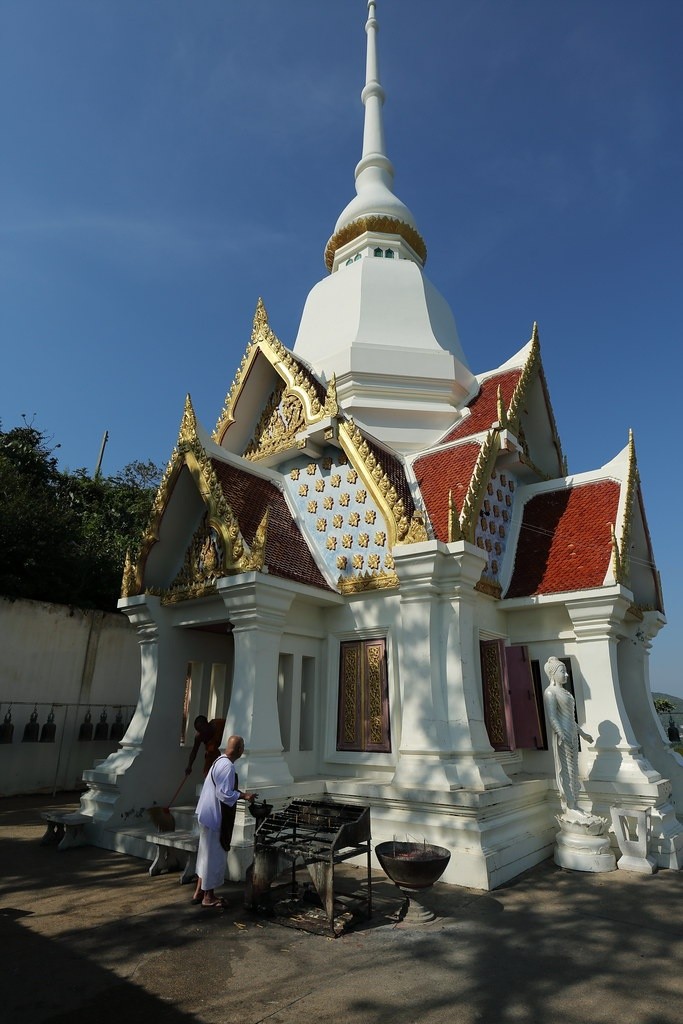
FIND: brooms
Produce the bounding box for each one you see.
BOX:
[145,775,188,832]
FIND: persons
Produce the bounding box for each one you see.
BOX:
[121,549,136,595]
[543,656,594,823]
[200,535,218,574]
[189,735,260,907]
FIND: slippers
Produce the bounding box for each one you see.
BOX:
[191,891,231,908]
